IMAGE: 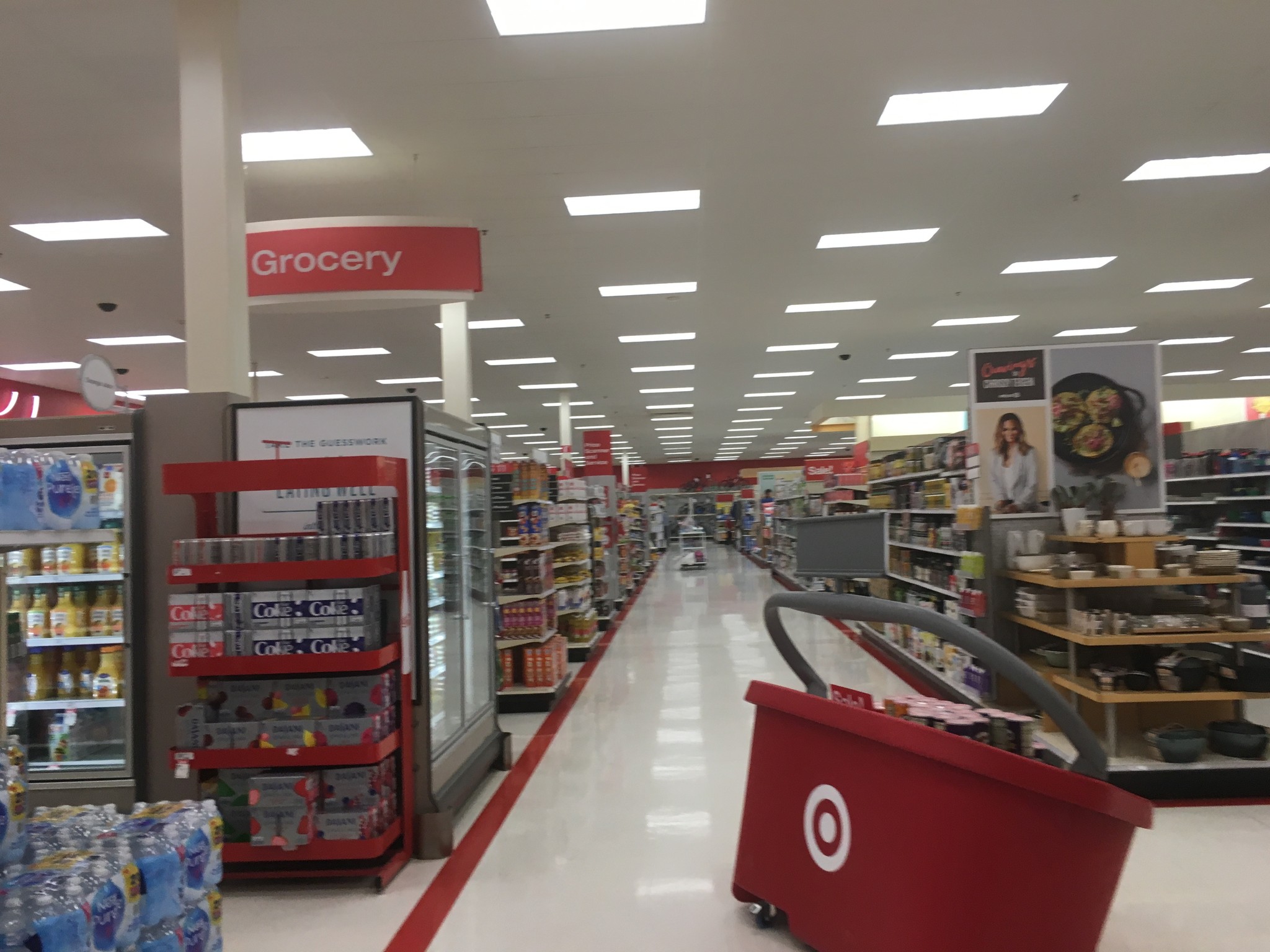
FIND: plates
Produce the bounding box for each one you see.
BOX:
[1194,550,1243,575]
[1153,596,1208,614]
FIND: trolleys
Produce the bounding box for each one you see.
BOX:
[679,517,708,570]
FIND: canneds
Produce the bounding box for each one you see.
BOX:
[316,497,396,535]
[173,530,395,565]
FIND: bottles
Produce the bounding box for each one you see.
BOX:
[48,714,69,763]
[92,645,125,699]
[0,734,225,952]
[5,585,124,639]
[0,542,125,576]
[23,646,101,702]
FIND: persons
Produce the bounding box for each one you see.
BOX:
[987,413,1046,514]
[761,488,774,503]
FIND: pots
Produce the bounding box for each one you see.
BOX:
[1052,373,1144,467]
[1011,554,1058,570]
[1036,645,1095,668]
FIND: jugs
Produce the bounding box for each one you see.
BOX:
[1006,530,1045,570]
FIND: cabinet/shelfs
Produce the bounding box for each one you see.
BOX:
[679,532,708,569]
[649,490,741,539]
[0,393,646,891]
[716,520,736,542]
[772,468,1270,772]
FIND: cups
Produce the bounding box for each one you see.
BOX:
[1124,521,1146,536]
[1076,520,1094,537]
[1147,520,1173,536]
[1125,452,1152,478]
[1096,520,1118,538]
[1060,507,1087,535]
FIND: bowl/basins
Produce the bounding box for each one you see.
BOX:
[1106,565,1133,578]
[1260,511,1270,524]
[1163,563,1191,577]
[1068,571,1095,579]
[1224,618,1250,632]
[1135,568,1161,578]
[1052,567,1072,579]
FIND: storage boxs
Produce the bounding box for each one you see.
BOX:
[834,473,862,484]
[825,490,855,500]
[741,489,754,498]
[804,458,855,480]
[869,495,896,507]
[715,495,734,509]
